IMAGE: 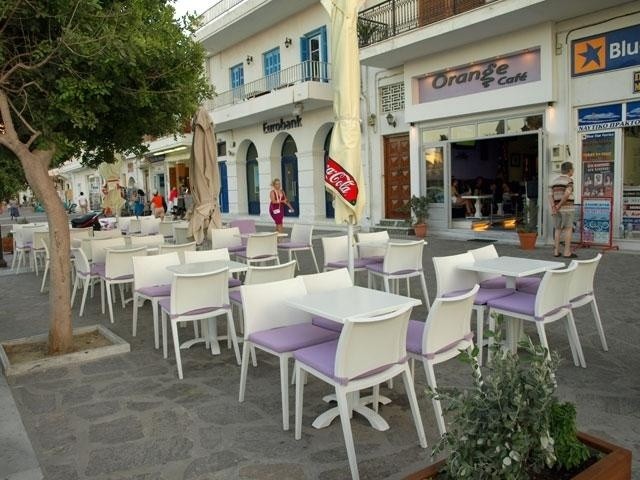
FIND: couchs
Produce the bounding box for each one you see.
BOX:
[427,178,477,217]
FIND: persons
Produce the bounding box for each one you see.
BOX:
[0,177,180,222]
[451,177,538,216]
[584,173,611,196]
[548,160,579,258]
[268,177,295,234]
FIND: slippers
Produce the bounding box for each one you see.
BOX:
[564,253,578,258]
[554,253,561,257]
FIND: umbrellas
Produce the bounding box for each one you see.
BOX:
[93,140,129,216]
[319,0,372,228]
[180,105,223,248]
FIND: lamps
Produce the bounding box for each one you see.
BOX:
[284,36,292,48]
[247,55,253,65]
[386,113,397,129]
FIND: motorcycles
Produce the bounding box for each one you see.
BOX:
[68,203,103,230]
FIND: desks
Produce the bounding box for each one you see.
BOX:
[461,193,495,219]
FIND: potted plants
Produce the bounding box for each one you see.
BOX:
[399,307,634,480]
[405,195,433,240]
[514,196,542,252]
[357,19,379,48]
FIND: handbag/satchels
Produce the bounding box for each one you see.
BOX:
[273,209,280,214]
